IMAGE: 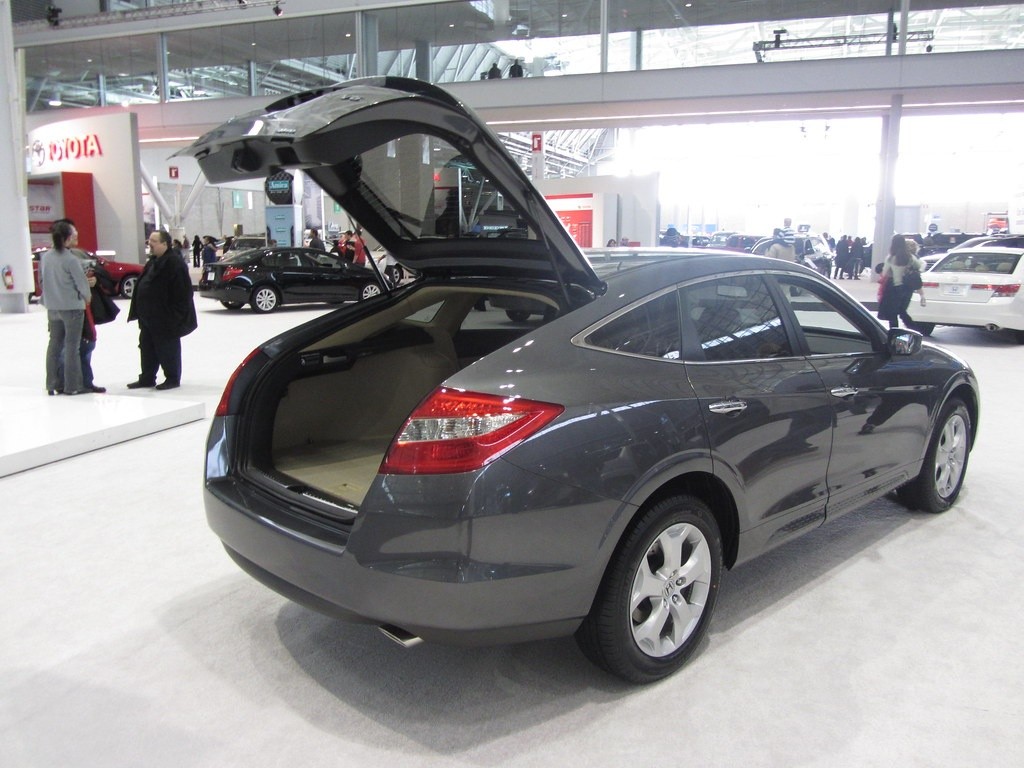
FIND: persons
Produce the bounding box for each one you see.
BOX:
[202,237,218,267]
[172,234,204,268]
[621,237,630,246]
[223,238,234,253]
[764,218,798,261]
[820,232,867,280]
[126,230,198,390]
[488,59,522,80]
[329,229,367,269]
[918,233,936,246]
[308,228,326,251]
[37,218,106,397]
[877,234,928,335]
[607,239,619,246]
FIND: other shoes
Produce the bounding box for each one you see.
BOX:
[48,384,106,395]
[155,380,180,390]
[127,381,156,389]
[920,297,926,307]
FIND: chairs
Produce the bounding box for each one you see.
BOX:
[695,306,759,358]
[951,261,966,270]
[975,264,990,272]
[997,263,1012,272]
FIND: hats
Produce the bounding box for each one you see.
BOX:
[667,228,680,236]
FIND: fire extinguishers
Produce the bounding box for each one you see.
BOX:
[5,264,14,290]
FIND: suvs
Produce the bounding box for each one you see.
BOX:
[221,235,267,257]
[744,231,832,296]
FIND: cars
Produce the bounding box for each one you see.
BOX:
[29,248,144,300]
[917,233,1024,273]
[167,70,981,684]
[303,239,338,256]
[906,247,1024,335]
[680,232,763,251]
[198,245,395,314]
[848,233,987,273]
[365,236,449,284]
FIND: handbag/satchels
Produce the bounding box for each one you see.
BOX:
[902,266,923,291]
